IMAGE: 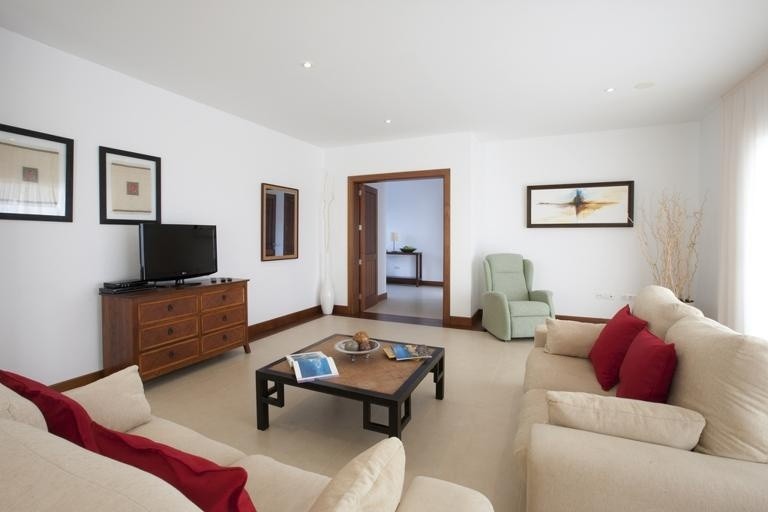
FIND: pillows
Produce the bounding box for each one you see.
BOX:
[91,420,255,511]
[308,437,407,512]
[61,364,152,433]
[0,370,100,451]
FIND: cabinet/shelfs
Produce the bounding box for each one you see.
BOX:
[99,278,252,381]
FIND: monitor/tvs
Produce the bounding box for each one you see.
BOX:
[138,224,217,288]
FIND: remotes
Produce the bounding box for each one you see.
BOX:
[221,278,226,282]
[210,278,217,283]
[227,278,232,281]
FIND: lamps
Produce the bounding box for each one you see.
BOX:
[390,231,400,252]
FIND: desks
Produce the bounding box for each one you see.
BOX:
[387,252,422,286]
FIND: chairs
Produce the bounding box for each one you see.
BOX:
[481,254,555,341]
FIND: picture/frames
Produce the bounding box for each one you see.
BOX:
[99,145,162,225]
[527,181,635,228]
[0,124,75,222]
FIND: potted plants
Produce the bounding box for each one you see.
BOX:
[626,189,711,307]
[320,168,334,315]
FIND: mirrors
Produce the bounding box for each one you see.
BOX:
[262,183,299,260]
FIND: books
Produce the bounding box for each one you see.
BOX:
[285,351,339,384]
[382,344,432,361]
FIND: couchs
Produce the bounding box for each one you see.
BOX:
[516,323,767,512]
[0,371,493,512]
